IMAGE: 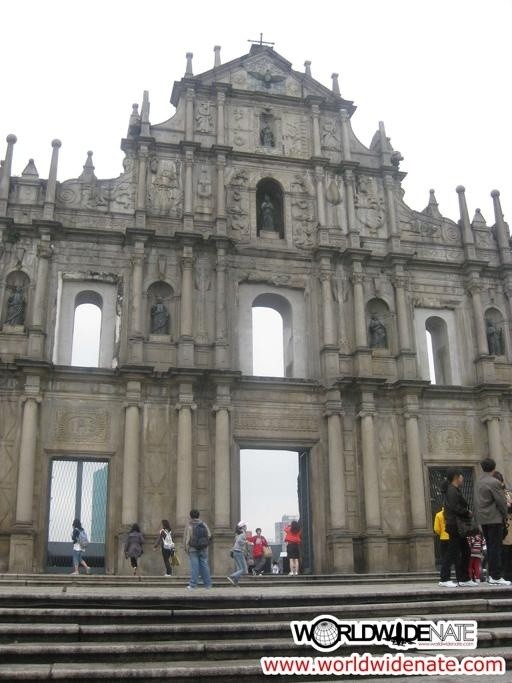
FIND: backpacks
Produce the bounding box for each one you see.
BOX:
[73,528,90,548]
[190,521,211,549]
[159,529,176,550]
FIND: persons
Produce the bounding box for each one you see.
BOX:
[183,510,211,591]
[487,320,501,355]
[369,315,386,348]
[474,458,511,586]
[261,195,274,231]
[153,520,175,576]
[150,297,169,334]
[433,506,449,569]
[124,524,145,576]
[260,123,273,147]
[72,520,91,575]
[439,467,479,587]
[494,471,512,581]
[227,521,304,587]
[5,286,27,324]
[467,528,488,584]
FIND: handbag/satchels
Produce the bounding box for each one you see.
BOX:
[229,550,234,558]
[171,555,181,565]
[456,517,480,538]
[263,546,273,558]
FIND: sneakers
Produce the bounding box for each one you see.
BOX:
[438,581,458,588]
[488,576,511,586]
[227,576,236,585]
[459,581,479,586]
[288,571,298,576]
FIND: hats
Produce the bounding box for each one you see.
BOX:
[237,520,246,528]
